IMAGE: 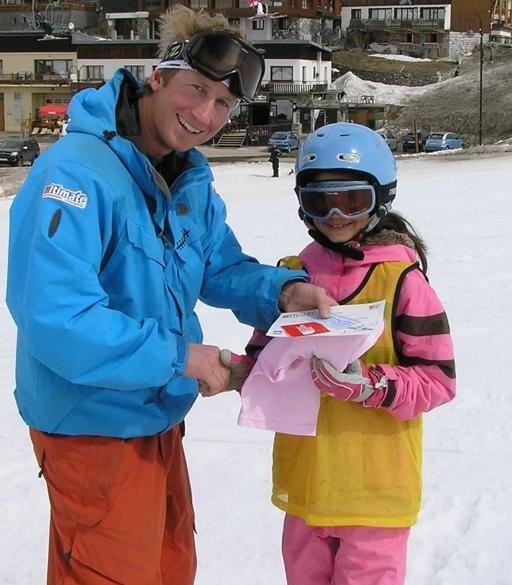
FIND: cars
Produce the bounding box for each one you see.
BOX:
[424,131,464,152]
[268,132,301,153]
[402,132,430,153]
[379,130,398,152]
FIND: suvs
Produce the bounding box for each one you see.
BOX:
[0,138,42,168]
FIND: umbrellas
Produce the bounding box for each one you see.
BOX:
[39,102,67,119]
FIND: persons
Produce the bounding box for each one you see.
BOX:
[48,115,69,135]
[268,141,281,177]
[2,2,343,585]
[34,10,54,35]
[197,120,456,585]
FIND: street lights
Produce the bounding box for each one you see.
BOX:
[465,13,496,144]
[64,64,91,95]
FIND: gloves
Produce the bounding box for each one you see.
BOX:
[309,354,387,411]
[197,346,256,396]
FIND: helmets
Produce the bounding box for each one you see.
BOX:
[295,121,397,203]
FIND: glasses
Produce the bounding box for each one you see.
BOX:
[160,31,267,102]
[296,179,377,218]
